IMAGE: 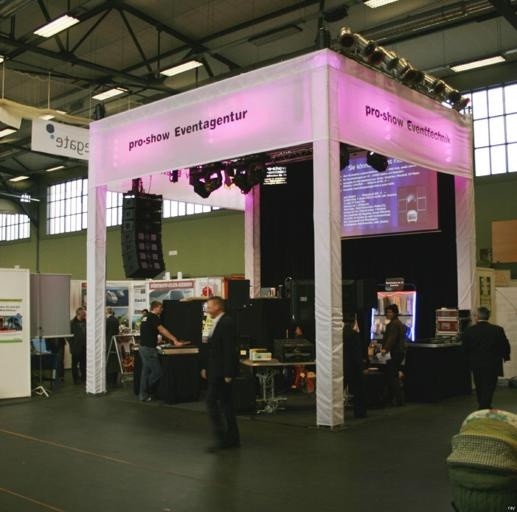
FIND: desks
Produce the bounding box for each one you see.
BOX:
[241,359,315,411]
[405,342,470,402]
[134,347,200,404]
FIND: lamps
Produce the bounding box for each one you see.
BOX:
[234,162,268,195]
[364,0,399,8]
[160,59,204,77]
[366,151,389,172]
[338,26,470,112]
[450,53,506,73]
[92,87,129,103]
[194,163,222,198]
[0,53,67,182]
[33,12,80,40]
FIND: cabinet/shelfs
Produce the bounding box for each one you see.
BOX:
[371,290,417,341]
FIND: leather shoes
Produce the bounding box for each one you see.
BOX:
[206,442,242,452]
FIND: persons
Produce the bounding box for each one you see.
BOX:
[105,307,119,352]
[137,301,192,402]
[66,308,86,384]
[380,304,406,407]
[44,336,66,385]
[199,296,242,451]
[459,307,511,410]
[343,311,370,419]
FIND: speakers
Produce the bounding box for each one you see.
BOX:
[122,193,167,280]
